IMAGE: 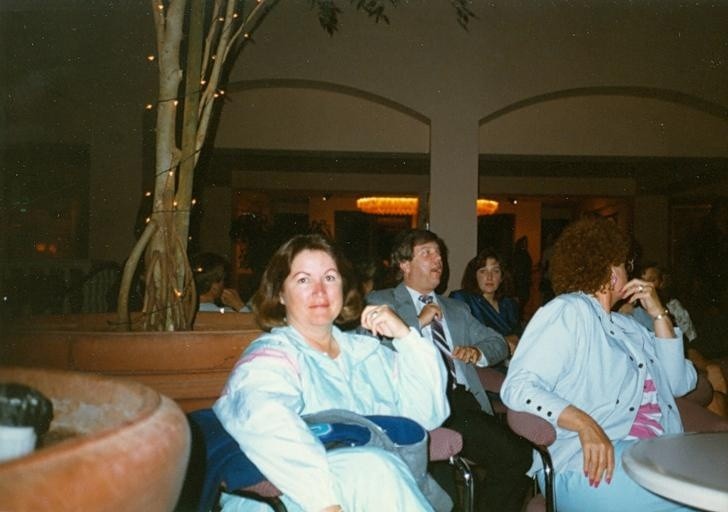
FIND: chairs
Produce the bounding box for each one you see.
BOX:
[454,368,537,512]
[212,428,458,512]
[58,265,122,312]
[506,397,727,512]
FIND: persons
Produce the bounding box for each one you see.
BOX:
[445,249,524,372]
[498,211,699,512]
[186,217,414,334]
[616,263,728,433]
[355,228,535,512]
[214,232,452,511]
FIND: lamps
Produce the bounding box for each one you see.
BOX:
[356,197,500,216]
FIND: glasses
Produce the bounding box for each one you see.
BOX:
[625,259,633,274]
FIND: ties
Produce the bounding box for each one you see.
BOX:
[419,294,457,386]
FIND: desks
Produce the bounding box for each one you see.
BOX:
[621,431,727,512]
